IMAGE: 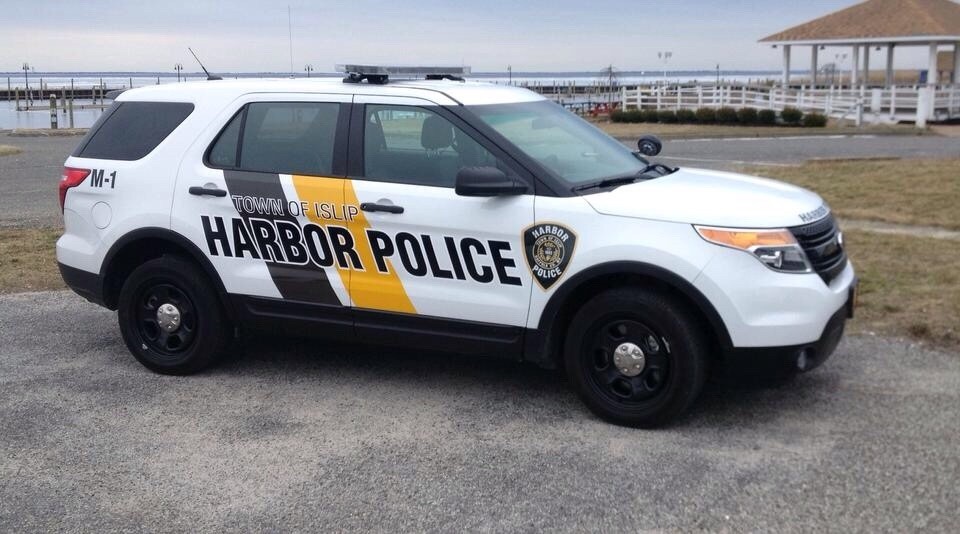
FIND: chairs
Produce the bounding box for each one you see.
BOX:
[421,117,457,186]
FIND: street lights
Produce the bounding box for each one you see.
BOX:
[304,63,313,77]
[174,63,183,82]
[22,62,30,89]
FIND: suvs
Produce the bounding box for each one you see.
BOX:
[55,69,861,429]
